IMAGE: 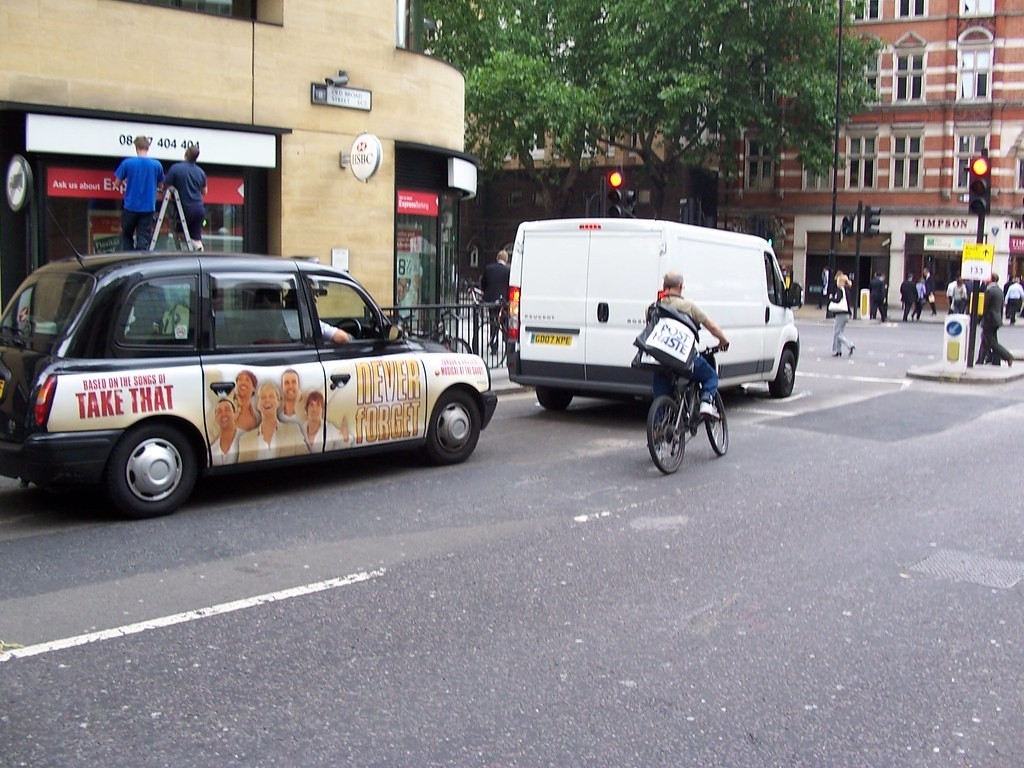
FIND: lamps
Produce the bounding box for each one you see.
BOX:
[325,70,349,86]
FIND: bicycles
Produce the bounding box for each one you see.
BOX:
[646,345,730,476]
[472,296,508,369]
[391,310,472,354]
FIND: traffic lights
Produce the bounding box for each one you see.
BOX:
[607,170,623,215]
[969,156,992,216]
[865,204,881,238]
[622,189,635,215]
[842,215,854,236]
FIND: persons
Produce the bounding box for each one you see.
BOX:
[291,279,354,346]
[645,270,729,462]
[947,272,1024,325]
[975,273,1015,368]
[870,271,889,324]
[900,268,936,322]
[114,136,165,251]
[164,146,208,252]
[481,250,510,356]
[816,264,845,310]
[780,263,790,297]
[211,369,353,466]
[828,273,855,357]
[398,278,411,305]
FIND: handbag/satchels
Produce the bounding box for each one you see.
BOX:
[828,286,848,312]
[928,292,935,303]
[629,295,700,380]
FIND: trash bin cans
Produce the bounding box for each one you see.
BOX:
[788,283,801,308]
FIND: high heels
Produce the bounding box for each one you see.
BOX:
[833,352,842,357]
[849,346,855,355]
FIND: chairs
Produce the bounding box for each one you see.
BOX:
[228,282,293,344]
[128,286,166,335]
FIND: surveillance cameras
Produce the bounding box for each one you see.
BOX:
[882,239,891,246]
[325,76,348,86]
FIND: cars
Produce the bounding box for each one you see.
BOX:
[0,250,498,516]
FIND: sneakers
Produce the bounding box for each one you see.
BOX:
[650,441,667,462]
[699,402,720,419]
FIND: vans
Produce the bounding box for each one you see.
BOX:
[507,216,804,409]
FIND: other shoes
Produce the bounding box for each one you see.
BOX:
[1008,356,1014,367]
